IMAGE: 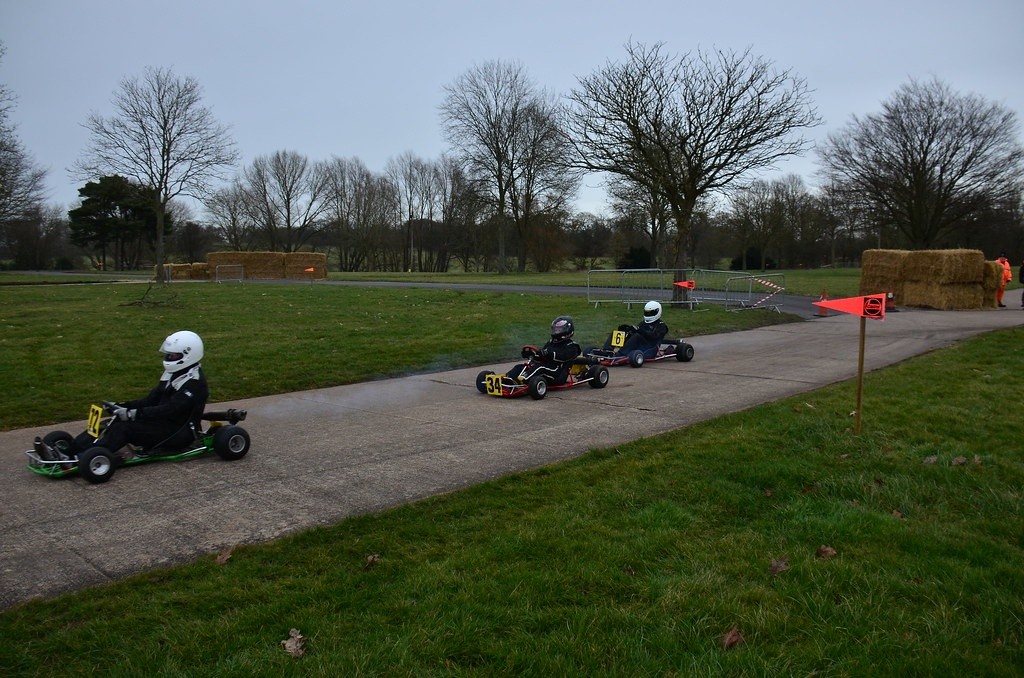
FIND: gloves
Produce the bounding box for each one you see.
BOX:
[103,403,118,411]
[630,324,636,332]
[113,408,135,422]
[521,350,534,359]
[617,325,623,331]
[534,351,548,361]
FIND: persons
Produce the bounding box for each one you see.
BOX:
[34,331,208,470]
[995,252,1012,307]
[602,301,668,359]
[502,316,581,386]
[1019,256,1024,308]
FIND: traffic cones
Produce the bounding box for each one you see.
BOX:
[884,286,900,312]
[812,289,834,318]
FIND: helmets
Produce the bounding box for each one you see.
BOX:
[643,301,663,324]
[159,331,204,374]
[550,317,574,342]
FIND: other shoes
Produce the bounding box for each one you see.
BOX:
[502,376,514,386]
[593,345,613,350]
[34,437,53,466]
[53,445,73,470]
